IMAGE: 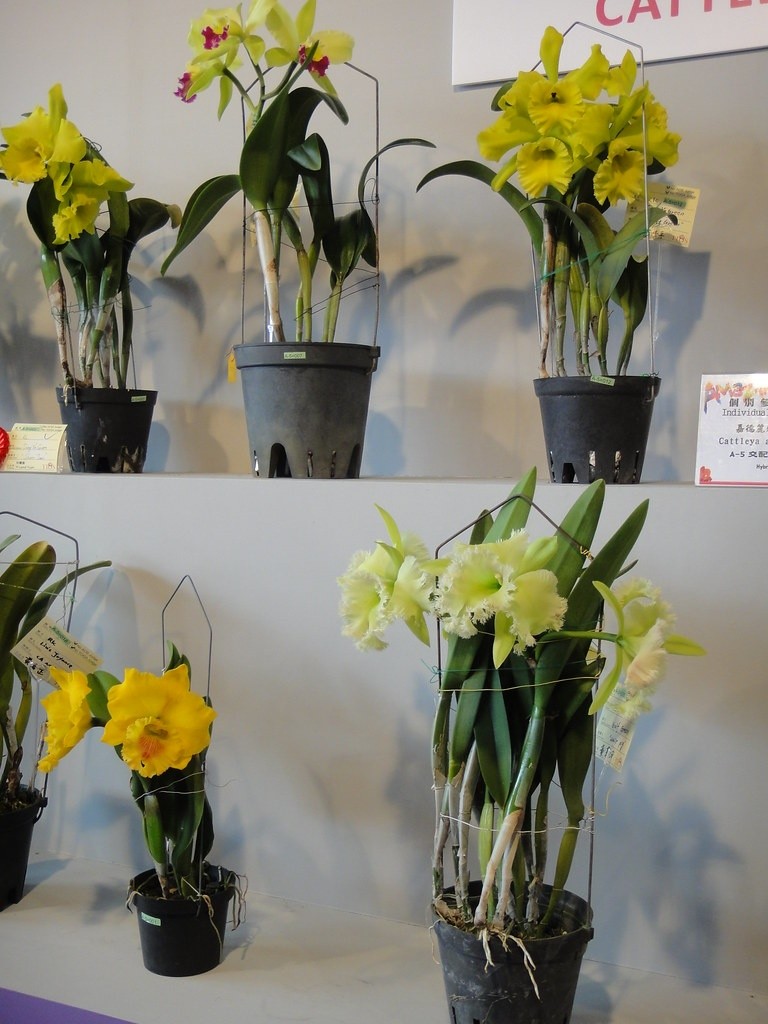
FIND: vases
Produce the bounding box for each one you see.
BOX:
[130,864,237,977]
[57,387,157,474]
[430,881,594,1024]
[232,340,381,479]
[533,377,660,486]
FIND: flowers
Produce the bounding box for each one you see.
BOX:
[415,27,679,377]
[336,467,704,939]
[0,84,182,388]
[161,1,436,341]
[37,645,217,898]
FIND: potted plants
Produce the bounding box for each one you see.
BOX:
[0,535,112,912]
